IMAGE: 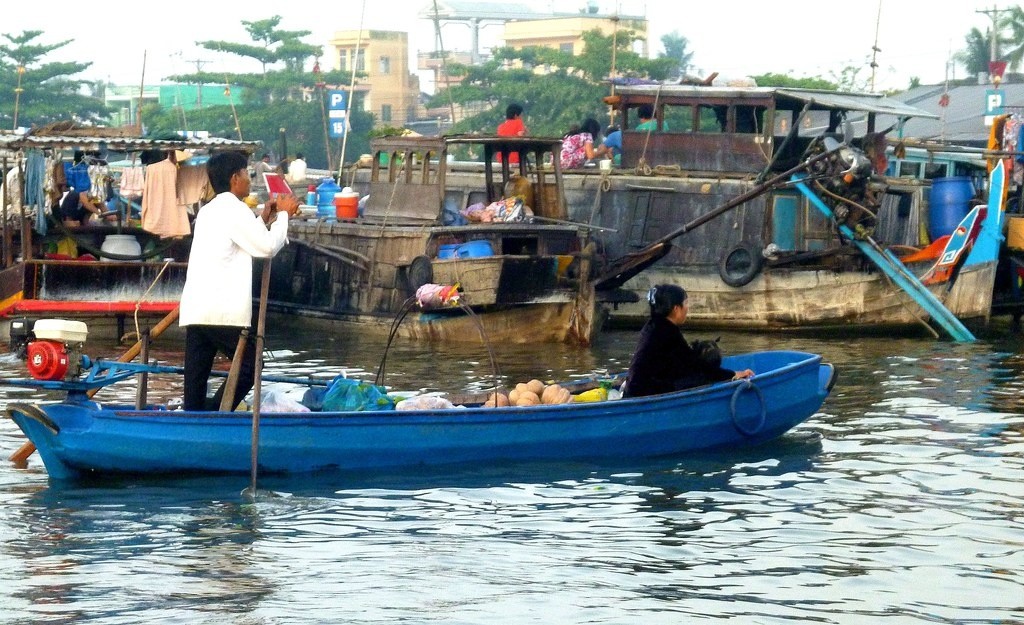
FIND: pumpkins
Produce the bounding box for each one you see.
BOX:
[481,379,573,407]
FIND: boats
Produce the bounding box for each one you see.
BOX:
[4,319,839,494]
[262,81,1024,333]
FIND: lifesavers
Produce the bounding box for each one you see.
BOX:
[719,242,762,287]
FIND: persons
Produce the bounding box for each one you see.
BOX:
[289,153,307,182]
[614,104,669,169]
[61,190,117,224]
[551,119,607,169]
[623,284,754,398]
[598,124,621,165]
[494,103,532,163]
[178,150,299,413]
[255,154,272,186]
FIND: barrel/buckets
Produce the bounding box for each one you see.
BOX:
[316,179,359,222]
[928,175,976,241]
[438,240,495,260]
[100,234,142,263]
